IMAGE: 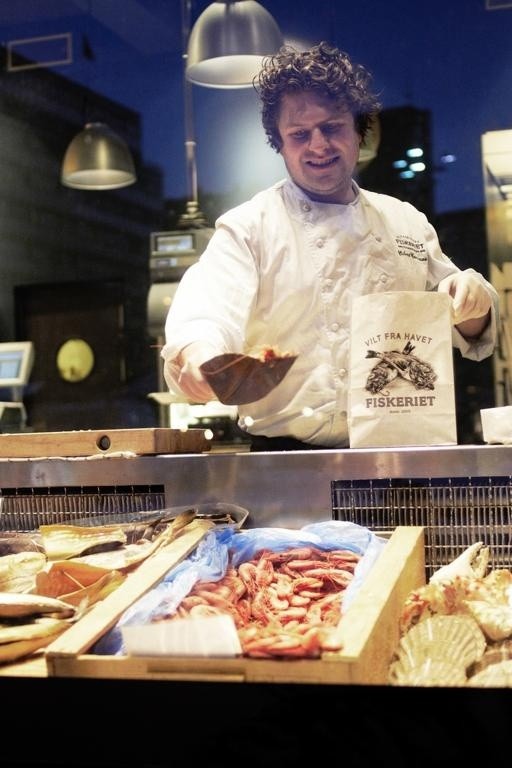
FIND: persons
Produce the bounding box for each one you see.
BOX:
[156,41,502,453]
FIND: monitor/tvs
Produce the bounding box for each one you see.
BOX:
[0,341,35,386]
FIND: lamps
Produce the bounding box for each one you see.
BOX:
[180,1,292,90]
[56,2,141,191]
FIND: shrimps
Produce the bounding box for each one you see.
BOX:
[148,546,362,660]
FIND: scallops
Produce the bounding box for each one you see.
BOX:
[388,540,512,687]
